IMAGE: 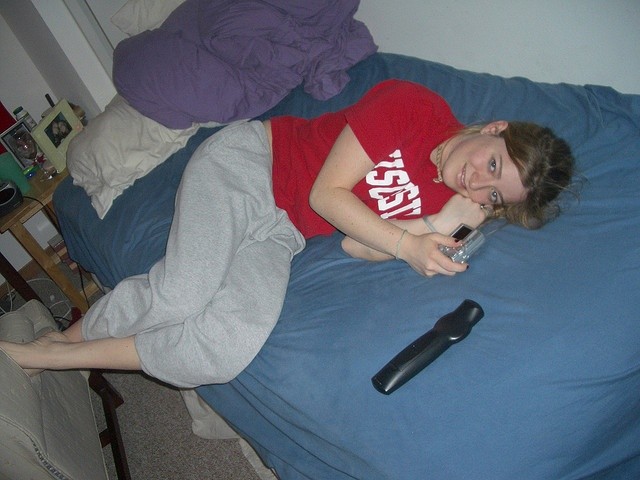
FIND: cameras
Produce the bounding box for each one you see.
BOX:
[439,223,486,264]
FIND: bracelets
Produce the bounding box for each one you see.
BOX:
[396,229,408,261]
[423,215,438,233]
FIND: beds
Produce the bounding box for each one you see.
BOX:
[53,51,639,478]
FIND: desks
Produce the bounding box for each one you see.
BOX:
[0,170,96,314]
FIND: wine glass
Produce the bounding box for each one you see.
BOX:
[8,130,55,181]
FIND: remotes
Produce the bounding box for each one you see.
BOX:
[371,298,485,395]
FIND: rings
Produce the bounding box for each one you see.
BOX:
[480,204,494,219]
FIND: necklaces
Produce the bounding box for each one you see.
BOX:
[433,135,457,183]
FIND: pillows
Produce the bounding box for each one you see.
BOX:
[66,96,198,220]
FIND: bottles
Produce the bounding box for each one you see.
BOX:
[13,106,37,132]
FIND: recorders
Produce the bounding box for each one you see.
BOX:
[0,180,23,217]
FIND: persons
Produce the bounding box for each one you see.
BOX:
[0,80,575,387]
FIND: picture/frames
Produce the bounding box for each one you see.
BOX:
[0,116,41,170]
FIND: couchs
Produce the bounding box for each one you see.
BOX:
[1,300,110,479]
